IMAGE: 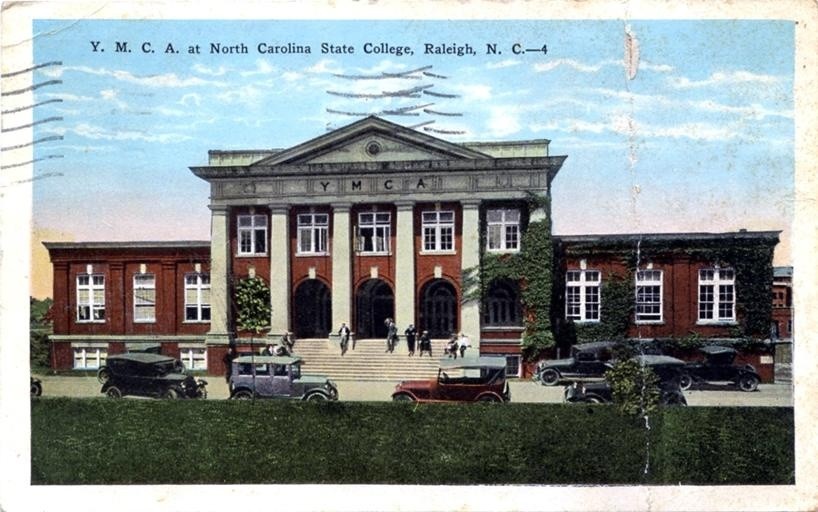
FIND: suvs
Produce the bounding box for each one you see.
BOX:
[561,354,688,406]
[225,353,339,403]
[30,374,43,397]
[99,348,208,401]
[386,354,512,403]
[679,344,762,392]
[529,335,624,388]
[94,342,164,385]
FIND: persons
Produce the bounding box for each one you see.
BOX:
[223,347,236,381]
[339,321,350,353]
[385,318,471,358]
[260,331,294,374]
